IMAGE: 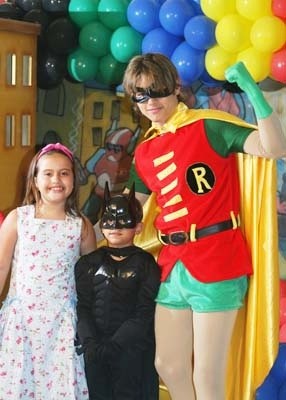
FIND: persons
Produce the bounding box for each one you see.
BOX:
[97,53,286,400]
[75,181,162,400]
[0,141,97,400]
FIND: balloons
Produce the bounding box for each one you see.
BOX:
[0,0,286,87]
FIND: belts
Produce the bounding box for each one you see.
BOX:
[158,216,238,246]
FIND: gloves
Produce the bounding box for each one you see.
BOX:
[224,61,273,119]
[76,338,124,381]
